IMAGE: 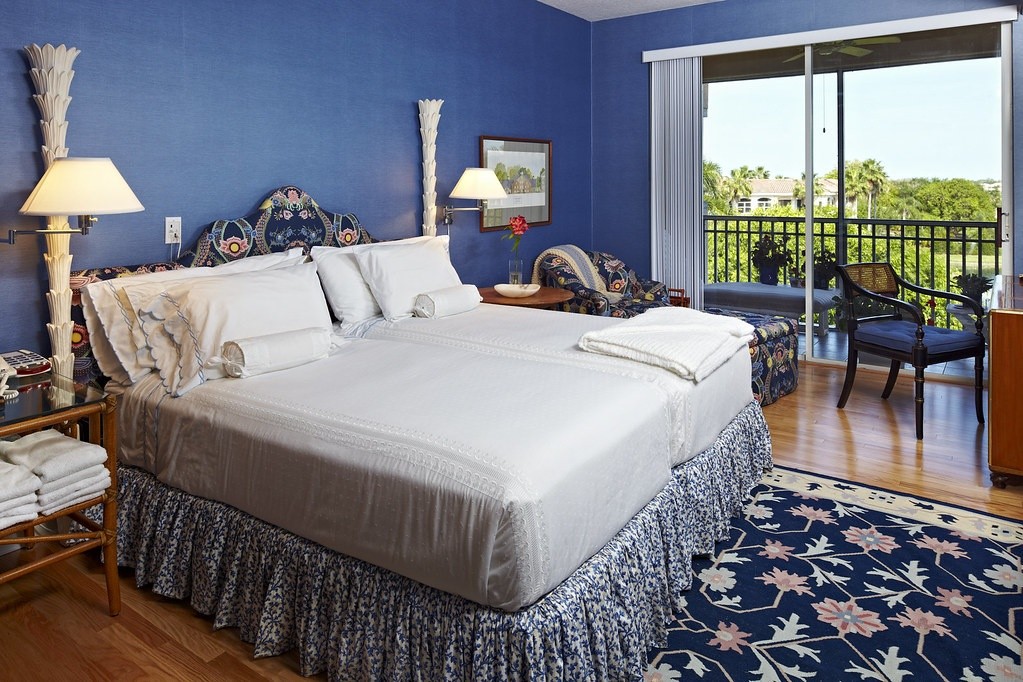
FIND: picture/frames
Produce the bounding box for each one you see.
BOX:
[479,136,553,231]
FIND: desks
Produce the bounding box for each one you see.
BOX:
[946,302,994,339]
[705,281,842,337]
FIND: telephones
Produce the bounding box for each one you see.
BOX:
[0,350,52,378]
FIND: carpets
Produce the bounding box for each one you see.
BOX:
[661,467,1023,682]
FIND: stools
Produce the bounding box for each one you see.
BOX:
[702,305,800,404]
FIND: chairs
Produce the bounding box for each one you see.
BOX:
[838,261,985,439]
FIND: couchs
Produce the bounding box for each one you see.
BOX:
[545,246,670,319]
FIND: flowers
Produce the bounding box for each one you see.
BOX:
[501,217,530,254]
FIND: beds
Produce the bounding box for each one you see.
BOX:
[68,187,774,682]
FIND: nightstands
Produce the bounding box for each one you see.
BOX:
[0,371,120,618]
[480,283,575,309]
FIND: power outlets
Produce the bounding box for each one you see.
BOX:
[166,217,182,246]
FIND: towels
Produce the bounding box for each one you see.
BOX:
[0,427,111,530]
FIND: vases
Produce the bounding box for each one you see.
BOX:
[509,260,523,291]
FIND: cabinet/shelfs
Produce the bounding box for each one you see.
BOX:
[988,274,1023,476]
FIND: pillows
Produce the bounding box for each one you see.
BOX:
[77,234,474,397]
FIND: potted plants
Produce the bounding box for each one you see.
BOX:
[787,267,804,287]
[832,294,858,332]
[952,273,992,308]
[801,248,839,290]
[750,234,793,286]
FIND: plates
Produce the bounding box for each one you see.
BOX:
[494,284,541,298]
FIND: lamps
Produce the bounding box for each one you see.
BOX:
[439,165,507,233]
[0,156,146,243]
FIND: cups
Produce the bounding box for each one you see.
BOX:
[508,259,522,284]
